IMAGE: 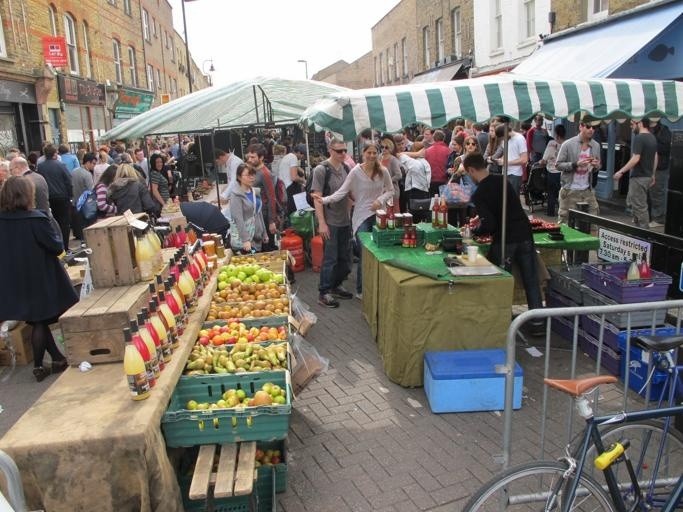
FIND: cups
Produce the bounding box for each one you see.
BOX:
[466,246,478,264]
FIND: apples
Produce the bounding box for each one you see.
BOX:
[196,254,289,345]
[186,448,281,476]
[187,382,286,431]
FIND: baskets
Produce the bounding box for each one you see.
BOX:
[180,438,287,511]
[543,260,683,401]
[160,248,292,449]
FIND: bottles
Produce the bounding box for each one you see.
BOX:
[121,225,211,401]
[626,253,639,280]
[460,217,474,239]
[375,202,416,248]
[431,194,439,227]
[638,253,650,279]
[438,195,447,228]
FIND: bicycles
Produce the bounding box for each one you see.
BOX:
[460,333,683,511]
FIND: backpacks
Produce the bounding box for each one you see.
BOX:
[305,160,350,210]
[77,189,97,219]
[275,176,288,215]
[397,166,406,191]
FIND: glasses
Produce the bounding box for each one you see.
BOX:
[331,147,347,154]
[466,142,477,147]
[582,124,596,130]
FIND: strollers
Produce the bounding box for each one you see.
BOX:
[176,199,230,246]
[520,159,551,214]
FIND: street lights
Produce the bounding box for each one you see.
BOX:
[202,58,215,86]
[297,58,309,81]
[180,0,207,94]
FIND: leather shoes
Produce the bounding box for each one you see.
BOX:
[31,360,68,383]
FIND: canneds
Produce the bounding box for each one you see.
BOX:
[202,233,225,270]
[376,210,413,229]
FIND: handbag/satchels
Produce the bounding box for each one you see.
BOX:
[229,218,256,250]
[408,197,431,214]
[439,173,472,210]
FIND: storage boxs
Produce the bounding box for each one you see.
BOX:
[57,285,154,370]
[82,213,148,287]
[542,261,682,401]
[422,348,523,414]
[0,319,37,370]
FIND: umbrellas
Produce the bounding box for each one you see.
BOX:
[297,70,683,272]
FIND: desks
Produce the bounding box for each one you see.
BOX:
[356,218,516,388]
[0,246,230,512]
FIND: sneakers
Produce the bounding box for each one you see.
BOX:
[316,292,337,308]
[329,284,353,300]
[648,219,665,228]
